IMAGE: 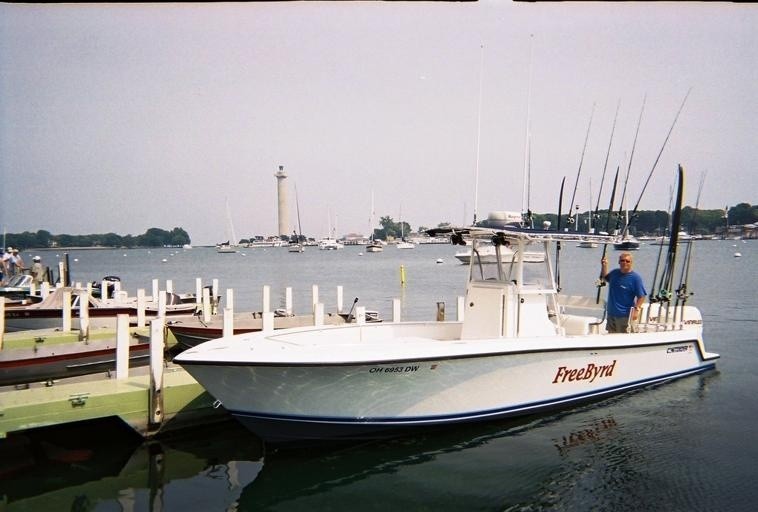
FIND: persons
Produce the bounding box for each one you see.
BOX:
[0,245,45,288]
[599,251,648,334]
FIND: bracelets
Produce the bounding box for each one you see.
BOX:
[634,306,641,311]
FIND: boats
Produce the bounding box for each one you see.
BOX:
[215,240,247,258]
[454,243,545,264]
[0,252,221,332]
[170,223,723,450]
[1,324,165,386]
[165,308,384,351]
[271,240,345,253]
[579,237,600,249]
[162,244,191,263]
[649,230,716,245]
[614,209,640,253]
[366,233,451,253]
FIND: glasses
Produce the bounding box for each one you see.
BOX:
[619,259,630,264]
